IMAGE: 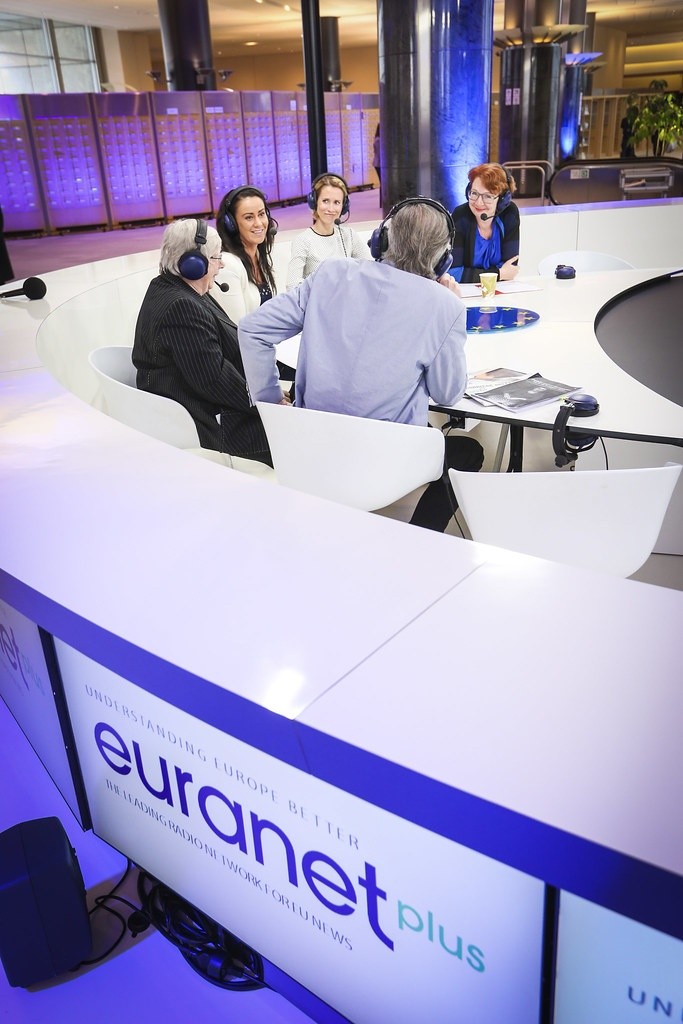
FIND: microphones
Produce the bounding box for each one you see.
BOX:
[335,211,349,225]
[0,276,46,301]
[270,218,279,235]
[480,206,506,221]
[214,280,229,292]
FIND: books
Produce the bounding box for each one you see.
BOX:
[463,367,583,413]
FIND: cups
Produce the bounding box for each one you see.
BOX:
[479,273,499,299]
[480,287,498,314]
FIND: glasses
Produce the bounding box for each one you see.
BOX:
[468,188,499,204]
[208,254,223,264]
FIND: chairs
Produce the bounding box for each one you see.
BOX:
[256,399,463,537]
[85,346,199,455]
[447,462,683,581]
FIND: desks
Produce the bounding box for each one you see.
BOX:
[261,251,683,468]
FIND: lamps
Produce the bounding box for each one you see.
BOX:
[194,66,233,86]
[296,82,306,91]
[330,79,353,89]
[145,71,172,85]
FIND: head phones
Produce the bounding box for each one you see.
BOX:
[465,166,513,214]
[555,264,576,279]
[222,185,266,236]
[178,218,209,280]
[307,173,350,215]
[367,197,456,278]
[553,393,600,468]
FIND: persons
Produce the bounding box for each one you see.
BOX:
[132,217,293,468]
[486,385,548,406]
[207,184,296,381]
[447,161,520,283]
[286,173,375,289]
[238,195,485,534]
[372,123,381,207]
